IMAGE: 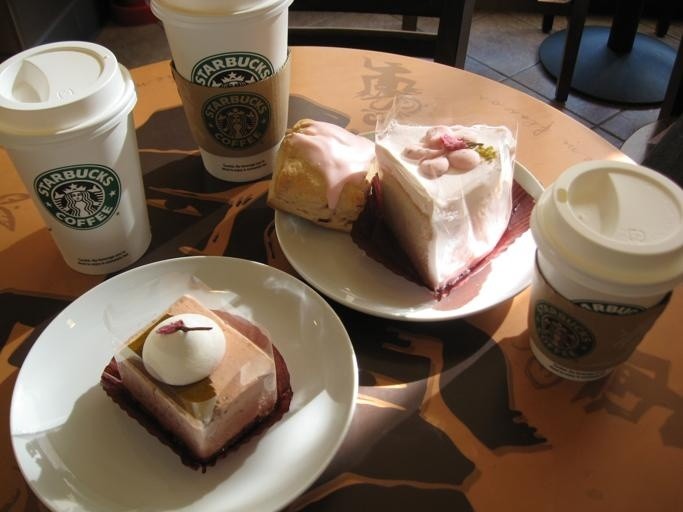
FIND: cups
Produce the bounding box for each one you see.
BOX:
[529,158,683,381]
[150,1,292,186]
[0,40,151,277]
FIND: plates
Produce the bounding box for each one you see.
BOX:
[10,255,359,512]
[275,130,544,320]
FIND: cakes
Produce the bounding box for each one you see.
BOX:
[98,295,293,474]
[267,119,379,232]
[374,123,535,304]
